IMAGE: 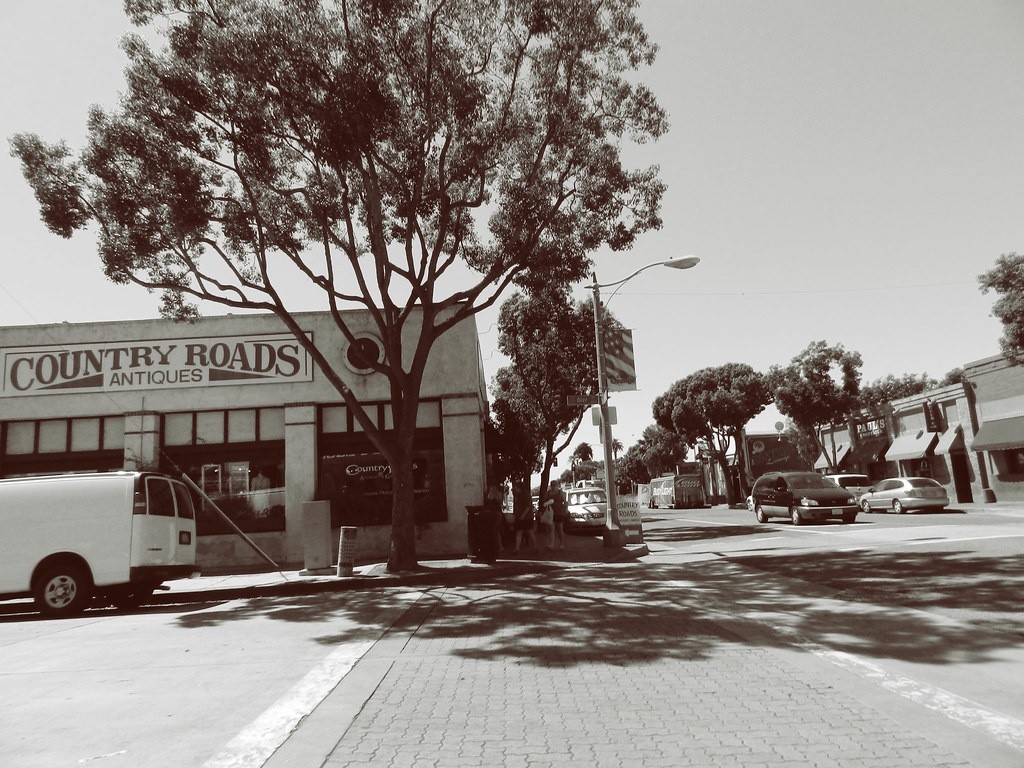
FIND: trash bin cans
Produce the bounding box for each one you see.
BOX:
[465,505,503,564]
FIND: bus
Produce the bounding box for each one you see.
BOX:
[576,479,606,491]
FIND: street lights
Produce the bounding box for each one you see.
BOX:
[586,254,700,546]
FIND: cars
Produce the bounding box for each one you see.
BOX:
[641,485,649,505]
[564,487,608,530]
[860,476,950,513]
[531,496,539,513]
[747,496,755,512]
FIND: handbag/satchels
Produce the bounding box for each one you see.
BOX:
[540,504,554,525]
[560,500,568,517]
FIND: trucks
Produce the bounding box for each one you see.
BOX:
[647,473,703,509]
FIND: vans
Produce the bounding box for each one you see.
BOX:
[751,470,860,525]
[1,472,197,618]
[823,474,874,508]
[197,463,284,524]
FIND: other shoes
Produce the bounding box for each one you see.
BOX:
[511,550,520,555]
[546,545,554,550]
[559,545,564,550]
[531,550,538,554]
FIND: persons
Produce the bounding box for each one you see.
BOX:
[512,482,539,554]
[486,485,504,552]
[543,480,567,550]
[249,473,271,519]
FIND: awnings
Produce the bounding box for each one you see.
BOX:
[933,425,961,455]
[884,431,936,461]
[845,437,889,464]
[970,416,1024,453]
[814,443,850,469]
